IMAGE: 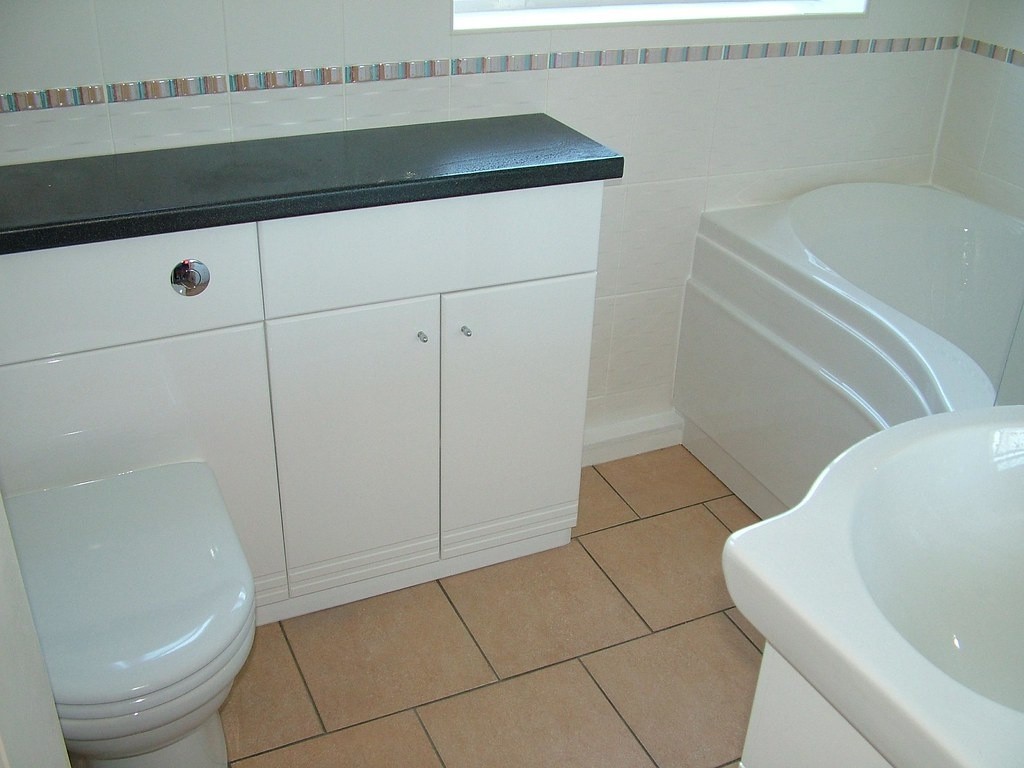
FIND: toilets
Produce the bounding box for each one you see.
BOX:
[0,459,262,767]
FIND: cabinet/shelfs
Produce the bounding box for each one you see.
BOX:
[0,218,300,680]
[256,176,609,611]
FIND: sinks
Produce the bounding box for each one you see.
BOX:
[717,403,1024,768]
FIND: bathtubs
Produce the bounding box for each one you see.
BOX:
[667,178,1023,522]
